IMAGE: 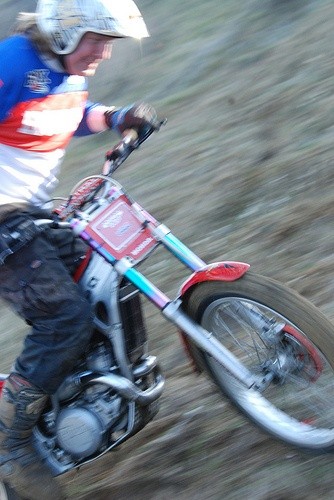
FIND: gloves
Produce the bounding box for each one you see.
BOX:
[103,103,158,136]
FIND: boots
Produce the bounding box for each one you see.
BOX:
[0,376,68,500]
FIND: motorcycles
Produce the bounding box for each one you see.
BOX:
[0,117,334,499]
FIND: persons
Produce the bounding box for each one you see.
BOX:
[0,0,152,500]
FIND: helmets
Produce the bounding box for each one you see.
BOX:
[34,0,152,55]
